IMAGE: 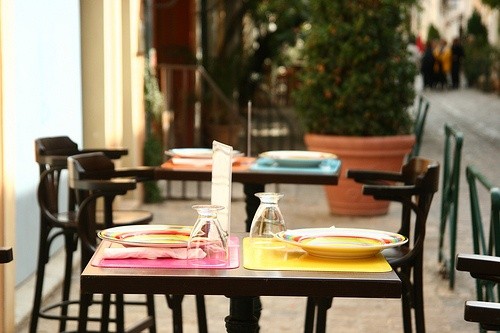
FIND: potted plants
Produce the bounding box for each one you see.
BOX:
[284,0,422,218]
[204,39,244,150]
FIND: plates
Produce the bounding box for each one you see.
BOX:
[164,148,240,158]
[258,150,338,165]
[274,227,409,260]
[96,225,230,248]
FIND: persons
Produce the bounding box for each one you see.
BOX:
[420,33,483,91]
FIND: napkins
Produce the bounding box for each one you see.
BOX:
[103,246,208,260]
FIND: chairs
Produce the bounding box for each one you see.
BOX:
[304,149,500,333]
[30,136,157,333]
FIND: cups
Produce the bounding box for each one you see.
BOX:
[187,204,228,265]
[250,192,287,237]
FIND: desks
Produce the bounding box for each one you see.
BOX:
[155,154,342,229]
[81,233,402,333]
